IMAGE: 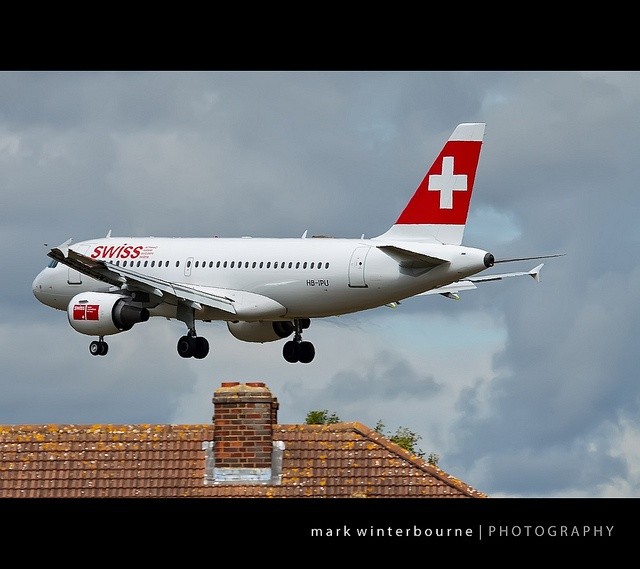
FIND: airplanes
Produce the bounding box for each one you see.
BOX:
[32,122,564,366]
